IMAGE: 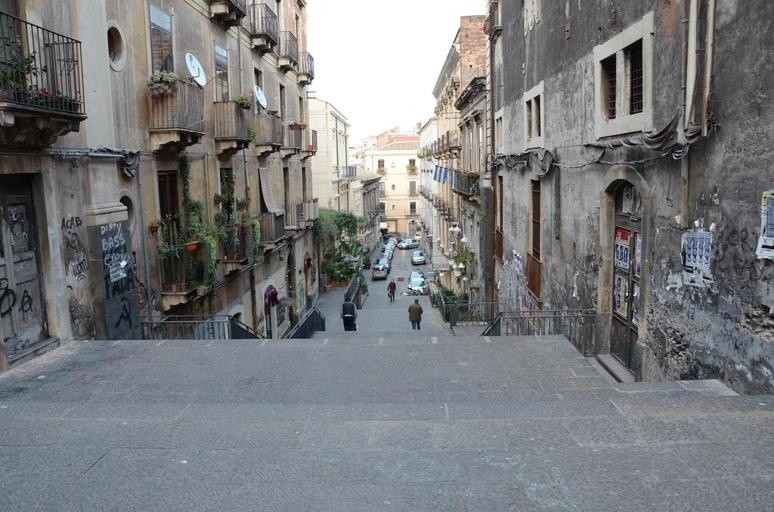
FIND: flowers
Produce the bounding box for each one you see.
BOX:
[146,70,178,101]
[233,91,255,107]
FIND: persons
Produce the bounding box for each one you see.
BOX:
[409,299,423,330]
[387,279,396,303]
[341,297,358,331]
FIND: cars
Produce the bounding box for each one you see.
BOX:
[399,239,417,250]
[372,240,395,281]
[401,271,428,296]
[411,251,427,264]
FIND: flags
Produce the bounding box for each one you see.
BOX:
[432,165,448,184]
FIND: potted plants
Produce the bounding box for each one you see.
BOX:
[148,220,160,232]
[323,243,337,293]
[183,236,198,251]
[176,262,191,292]
[0,39,48,102]
